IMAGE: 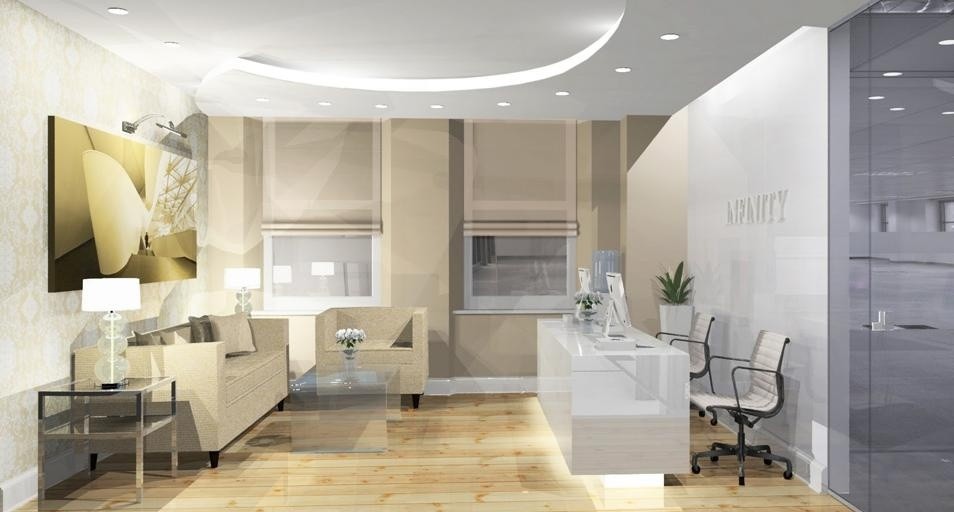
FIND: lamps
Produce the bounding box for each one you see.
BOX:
[81,277,140,386]
[121,113,187,138]
[273,260,335,295]
[223,267,261,312]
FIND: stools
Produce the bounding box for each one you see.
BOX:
[289,365,400,454]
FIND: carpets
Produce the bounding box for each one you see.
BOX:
[664,474,682,485]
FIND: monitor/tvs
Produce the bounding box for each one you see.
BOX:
[602,272,632,340]
[576,268,591,323]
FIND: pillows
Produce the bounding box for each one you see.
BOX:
[134,330,161,346]
[188,315,251,356]
[161,332,186,343]
[208,312,256,354]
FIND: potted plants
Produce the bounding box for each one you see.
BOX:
[655,261,694,353]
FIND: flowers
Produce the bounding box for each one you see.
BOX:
[575,290,603,308]
[336,328,366,346]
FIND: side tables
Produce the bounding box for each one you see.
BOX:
[37,377,178,503]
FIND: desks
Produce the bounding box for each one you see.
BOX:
[536,317,691,476]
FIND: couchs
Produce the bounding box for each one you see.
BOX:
[70,314,289,471]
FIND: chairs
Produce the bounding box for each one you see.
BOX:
[655,313,715,393]
[689,330,792,486]
[314,307,429,409]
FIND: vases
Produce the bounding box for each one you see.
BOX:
[344,347,358,361]
[579,308,597,320]
